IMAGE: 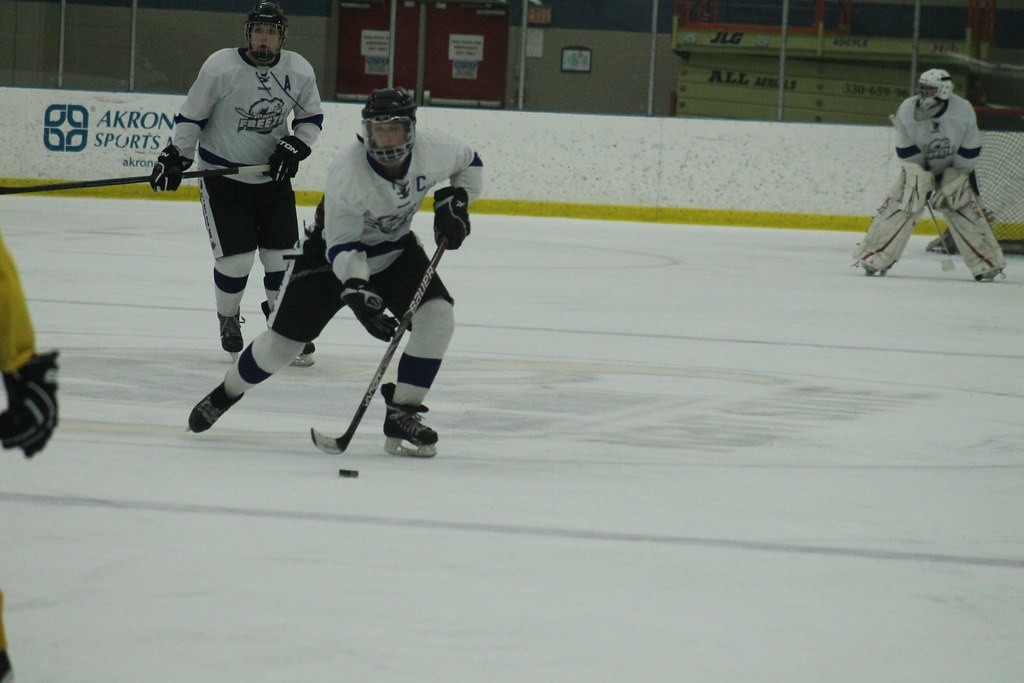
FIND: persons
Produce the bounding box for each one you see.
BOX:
[189,89,483,445]
[150,2,326,355]
[0,233,60,683]
[857,70,1008,280]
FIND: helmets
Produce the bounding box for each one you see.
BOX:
[244,0,289,63]
[362,88,417,168]
[916,69,954,110]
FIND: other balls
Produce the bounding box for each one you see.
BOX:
[339,468,360,479]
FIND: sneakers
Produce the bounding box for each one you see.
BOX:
[185,381,244,433]
[217,306,245,361]
[261,300,315,367]
[381,382,438,457]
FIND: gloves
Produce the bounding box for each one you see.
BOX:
[340,278,399,342]
[263,135,312,181]
[150,144,194,192]
[0,348,59,457]
[432,186,471,250]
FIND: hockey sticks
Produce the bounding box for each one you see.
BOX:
[308,238,450,457]
[1,163,272,199]
[887,113,958,275]
